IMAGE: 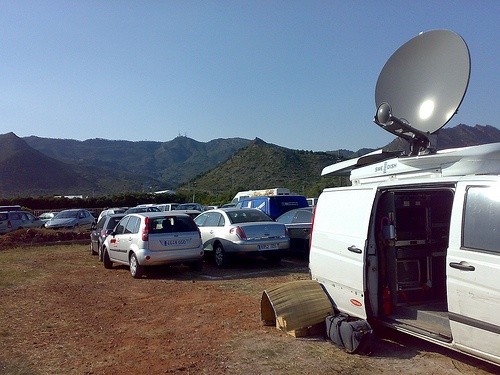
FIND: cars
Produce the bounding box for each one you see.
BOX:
[0,211,40,236]
[37,212,61,224]
[44,209,95,232]
[272,206,315,248]
[102,211,204,279]
[194,207,292,269]
[125,206,162,218]
[89,213,130,262]
[136,202,238,219]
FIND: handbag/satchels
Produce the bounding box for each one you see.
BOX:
[326,312,374,353]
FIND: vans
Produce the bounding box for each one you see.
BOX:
[306,197,319,209]
[307,143,500,371]
[0,205,23,212]
[234,195,310,221]
[231,188,291,208]
[93,208,127,226]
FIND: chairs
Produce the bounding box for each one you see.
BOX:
[151,221,158,232]
[162,220,175,232]
